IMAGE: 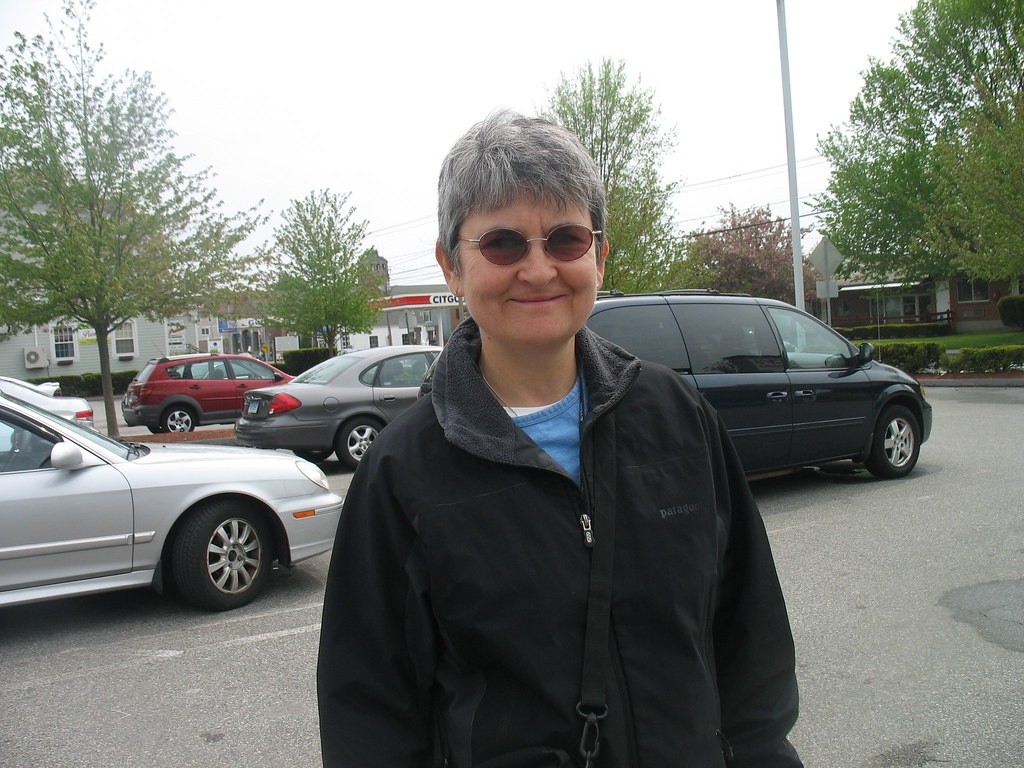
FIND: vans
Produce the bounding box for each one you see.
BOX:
[584,290,934,480]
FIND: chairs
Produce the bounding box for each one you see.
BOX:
[214,369,224,380]
[382,360,426,387]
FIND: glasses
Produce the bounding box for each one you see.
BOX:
[457,223,603,266]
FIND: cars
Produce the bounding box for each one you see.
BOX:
[0,391,345,613]
[121,353,297,434]
[233,345,443,472]
[0,376,101,434]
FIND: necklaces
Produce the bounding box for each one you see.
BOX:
[476,361,519,416]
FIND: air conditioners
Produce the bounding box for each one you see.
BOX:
[24,347,47,369]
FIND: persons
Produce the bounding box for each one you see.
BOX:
[316,109,805,768]
